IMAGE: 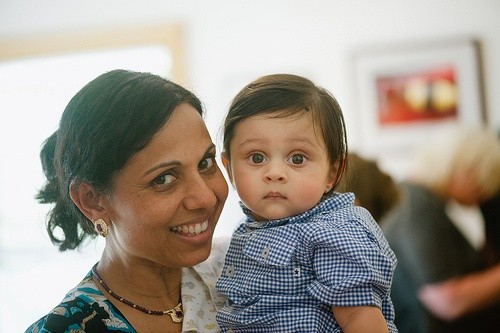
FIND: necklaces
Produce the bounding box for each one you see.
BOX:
[91,267,184,323]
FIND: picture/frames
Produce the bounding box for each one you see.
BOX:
[359,38,487,141]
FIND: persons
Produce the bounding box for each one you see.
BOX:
[25,69,235,333]
[333,128,500,333]
[221,74,399,333]
